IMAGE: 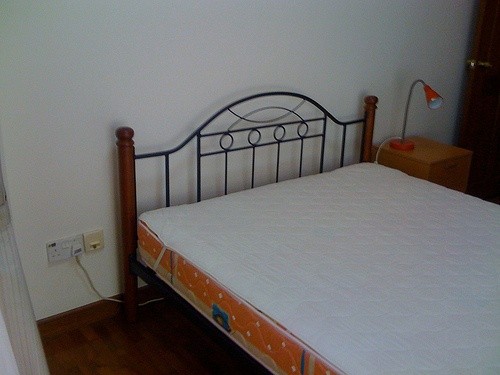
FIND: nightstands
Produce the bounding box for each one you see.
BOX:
[372,135,473,194]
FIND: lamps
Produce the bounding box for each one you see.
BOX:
[389,79,443,151]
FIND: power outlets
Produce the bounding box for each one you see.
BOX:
[46,233,83,264]
[84,229,105,254]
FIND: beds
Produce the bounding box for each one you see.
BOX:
[115,91,500,375]
